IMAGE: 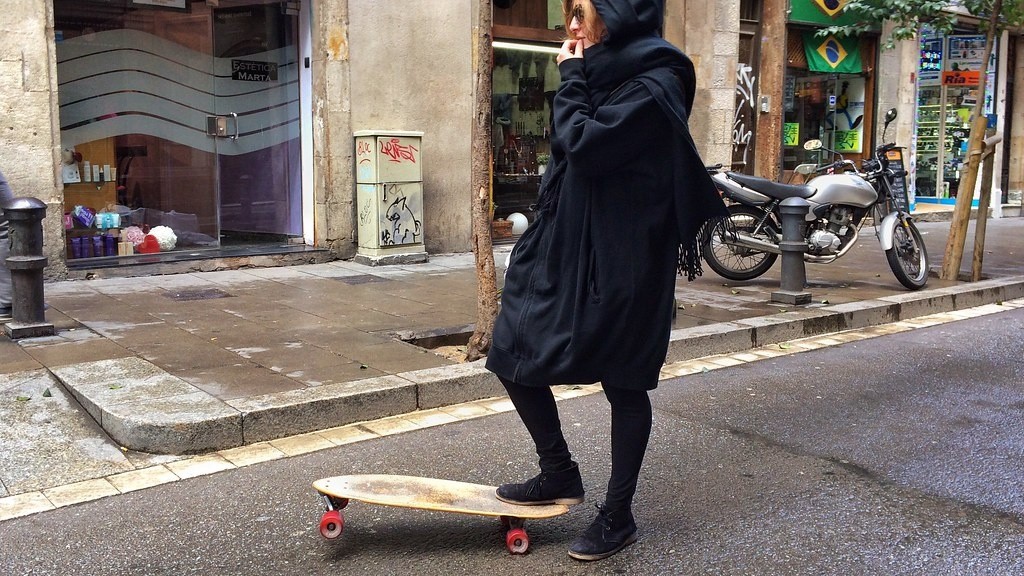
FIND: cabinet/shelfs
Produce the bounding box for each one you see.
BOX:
[917,34,996,204]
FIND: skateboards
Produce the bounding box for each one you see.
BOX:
[310,472,571,557]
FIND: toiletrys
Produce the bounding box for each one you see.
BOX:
[82,161,117,182]
[70,232,116,257]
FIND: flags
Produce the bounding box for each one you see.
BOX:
[801,29,863,74]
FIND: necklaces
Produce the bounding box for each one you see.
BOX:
[512,139,523,158]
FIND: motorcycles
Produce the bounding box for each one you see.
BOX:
[702,109,931,286]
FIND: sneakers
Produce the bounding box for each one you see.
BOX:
[567,503,638,560]
[495,461,586,505]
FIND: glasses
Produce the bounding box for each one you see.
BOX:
[568,3,585,25]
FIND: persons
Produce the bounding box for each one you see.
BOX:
[119,152,145,222]
[0,172,48,324]
[482,0,740,560]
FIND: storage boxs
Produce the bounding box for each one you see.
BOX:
[491,219,514,237]
